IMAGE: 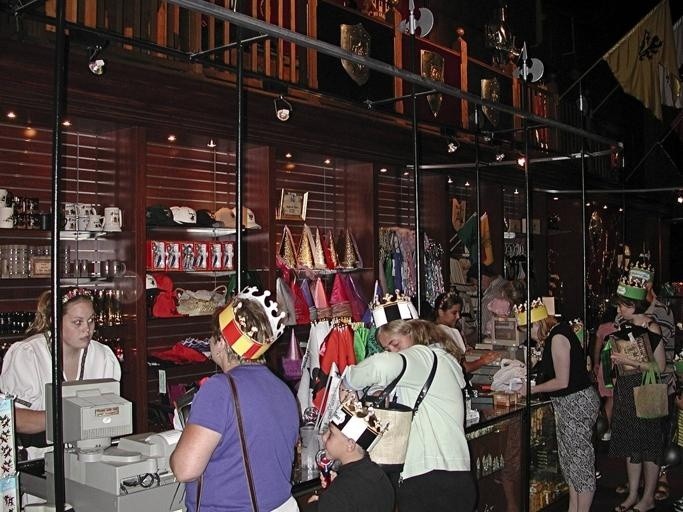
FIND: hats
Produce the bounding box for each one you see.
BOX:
[276,274,364,326]
[512,296,548,326]
[146,202,261,229]
[276,225,365,269]
[218,285,286,360]
[616,275,647,301]
[279,327,304,378]
[145,274,165,296]
[627,260,655,282]
[328,393,390,452]
[367,288,419,329]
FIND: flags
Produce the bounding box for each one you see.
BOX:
[604,0,681,123]
[664,17,683,109]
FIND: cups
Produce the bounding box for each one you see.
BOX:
[0,186,129,280]
[299,425,321,470]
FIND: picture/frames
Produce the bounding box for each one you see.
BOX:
[277,186,309,225]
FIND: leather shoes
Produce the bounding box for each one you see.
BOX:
[613,502,656,512]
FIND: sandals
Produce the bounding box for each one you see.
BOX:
[616,480,641,495]
[653,473,670,501]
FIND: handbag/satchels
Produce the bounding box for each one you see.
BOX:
[356,395,415,488]
[632,360,670,420]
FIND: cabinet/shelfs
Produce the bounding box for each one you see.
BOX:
[466,386,601,512]
[273,266,373,341]
[144,224,247,423]
[0,222,122,375]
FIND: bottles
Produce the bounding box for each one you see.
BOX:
[0,310,39,361]
[476,453,505,473]
[81,287,127,364]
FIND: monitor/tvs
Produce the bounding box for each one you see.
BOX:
[491,315,527,346]
[45,379,132,446]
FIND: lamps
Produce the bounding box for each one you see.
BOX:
[87,42,527,169]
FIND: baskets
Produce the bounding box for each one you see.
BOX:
[174,284,228,317]
